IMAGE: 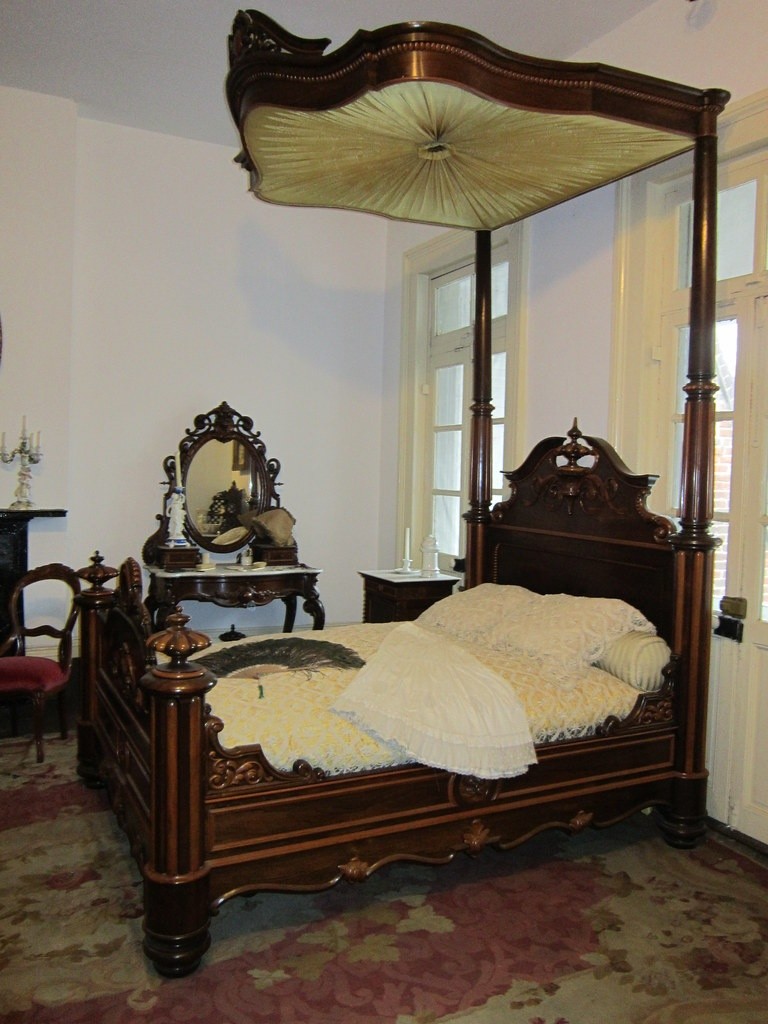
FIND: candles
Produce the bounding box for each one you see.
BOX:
[0,415,42,457]
[404,527,409,560]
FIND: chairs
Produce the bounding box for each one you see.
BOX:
[0,563,81,764]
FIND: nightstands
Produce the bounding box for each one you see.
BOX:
[360,569,461,623]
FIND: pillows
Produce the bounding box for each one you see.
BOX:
[489,594,656,690]
[416,583,542,646]
[594,630,672,694]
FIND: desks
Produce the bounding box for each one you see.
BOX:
[144,562,325,634]
[1,509,66,656]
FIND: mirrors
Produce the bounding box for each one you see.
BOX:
[177,430,267,555]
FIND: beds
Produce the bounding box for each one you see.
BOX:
[76,622,707,979]
